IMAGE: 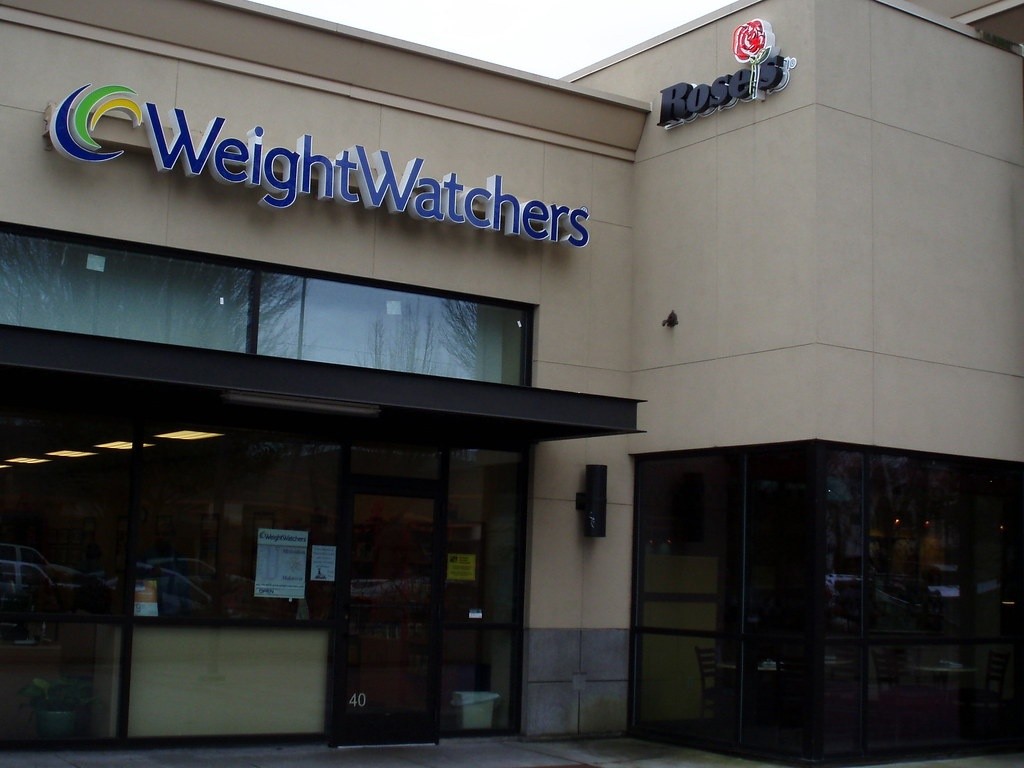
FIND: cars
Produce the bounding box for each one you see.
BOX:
[1,543,445,616]
[824,562,1003,625]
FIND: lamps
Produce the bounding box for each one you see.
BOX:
[575,464,607,537]
[220,389,381,419]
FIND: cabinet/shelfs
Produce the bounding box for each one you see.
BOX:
[345,520,486,713]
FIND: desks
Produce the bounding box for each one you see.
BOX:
[897,666,979,740]
[716,661,777,670]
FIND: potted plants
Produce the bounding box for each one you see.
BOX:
[19,676,96,739]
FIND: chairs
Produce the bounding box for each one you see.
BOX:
[869,647,919,742]
[776,660,813,747]
[693,644,738,736]
[959,648,1011,740]
